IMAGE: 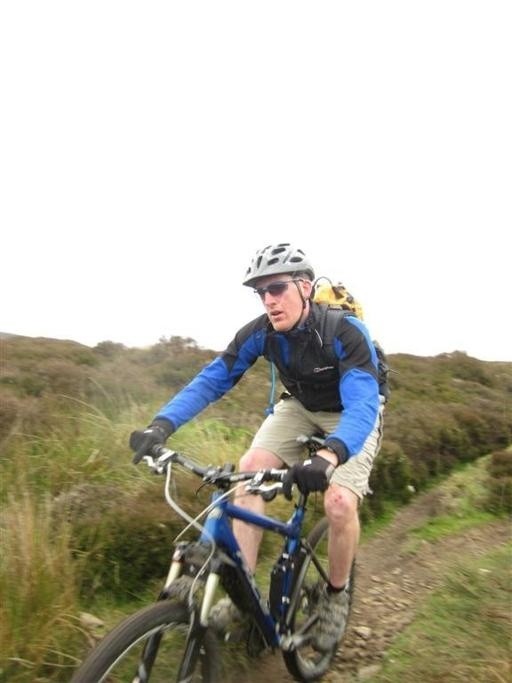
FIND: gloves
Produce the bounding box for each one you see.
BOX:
[283,454,335,502]
[129,418,175,466]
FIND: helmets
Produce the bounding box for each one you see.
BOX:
[243,240,314,288]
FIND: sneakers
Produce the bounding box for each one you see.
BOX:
[210,574,260,633]
[311,581,350,653]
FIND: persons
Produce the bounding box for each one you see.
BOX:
[128,240,389,655]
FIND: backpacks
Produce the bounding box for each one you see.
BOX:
[310,276,365,321]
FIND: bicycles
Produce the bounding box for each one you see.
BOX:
[69,432,356,683]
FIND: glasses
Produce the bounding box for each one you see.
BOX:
[253,279,302,303]
